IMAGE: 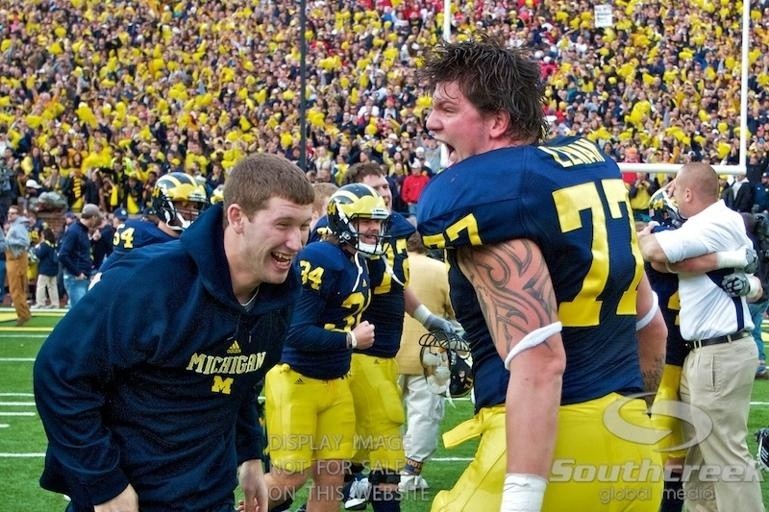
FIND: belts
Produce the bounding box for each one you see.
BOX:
[681,328,754,351]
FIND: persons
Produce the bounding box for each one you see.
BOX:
[0,1,769,510]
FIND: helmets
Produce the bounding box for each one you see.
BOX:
[326,182,392,259]
[648,188,686,230]
[418,330,474,398]
[152,172,209,234]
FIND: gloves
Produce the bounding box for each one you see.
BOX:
[722,270,751,298]
[421,314,457,340]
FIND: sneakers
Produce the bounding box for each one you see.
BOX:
[30,304,60,310]
[398,470,429,493]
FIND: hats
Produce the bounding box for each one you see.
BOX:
[82,203,105,220]
[114,207,128,221]
